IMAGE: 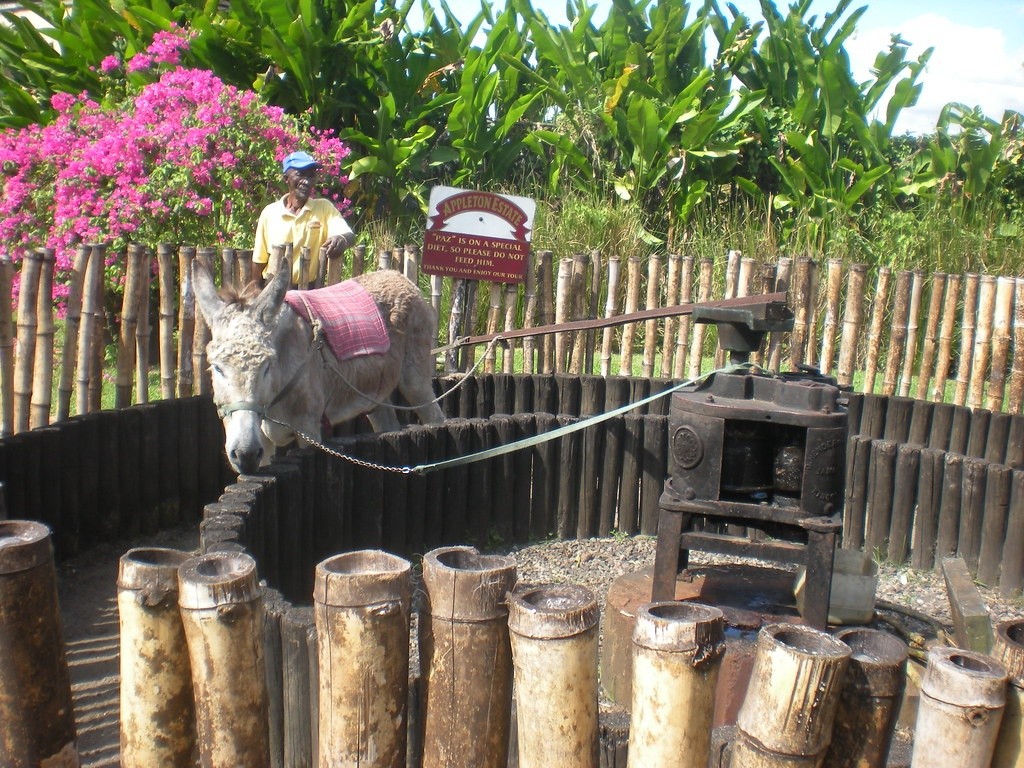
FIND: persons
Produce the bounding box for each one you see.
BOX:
[250,150,357,291]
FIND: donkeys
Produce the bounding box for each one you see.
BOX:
[191,258,448,475]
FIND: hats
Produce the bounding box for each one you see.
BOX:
[283,151,323,174]
